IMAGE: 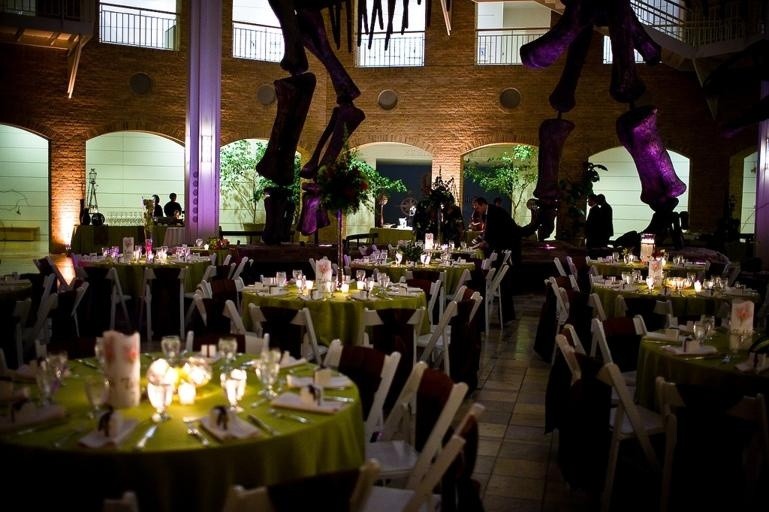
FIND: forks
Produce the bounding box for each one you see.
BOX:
[187,418,209,446]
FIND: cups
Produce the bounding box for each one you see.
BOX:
[362,250,466,265]
[298,385,323,408]
[89,252,98,258]
[415,240,466,251]
[314,370,332,387]
[598,251,684,266]
[98,410,123,438]
[607,269,745,296]
[101,243,200,263]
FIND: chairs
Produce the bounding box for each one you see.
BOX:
[324,339,485,512]
[2,225,222,349]
[545,251,768,512]
[222,238,510,384]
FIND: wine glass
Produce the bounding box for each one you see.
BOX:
[665,313,715,353]
[262,269,408,299]
[0,332,282,430]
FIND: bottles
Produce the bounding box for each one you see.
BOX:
[105,211,143,226]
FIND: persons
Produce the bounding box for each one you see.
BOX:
[153,194,163,216]
[411,191,613,248]
[172,207,183,225]
[164,193,182,217]
[475,197,522,326]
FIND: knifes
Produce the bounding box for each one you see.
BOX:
[136,425,158,448]
[248,414,278,436]
[269,407,307,424]
